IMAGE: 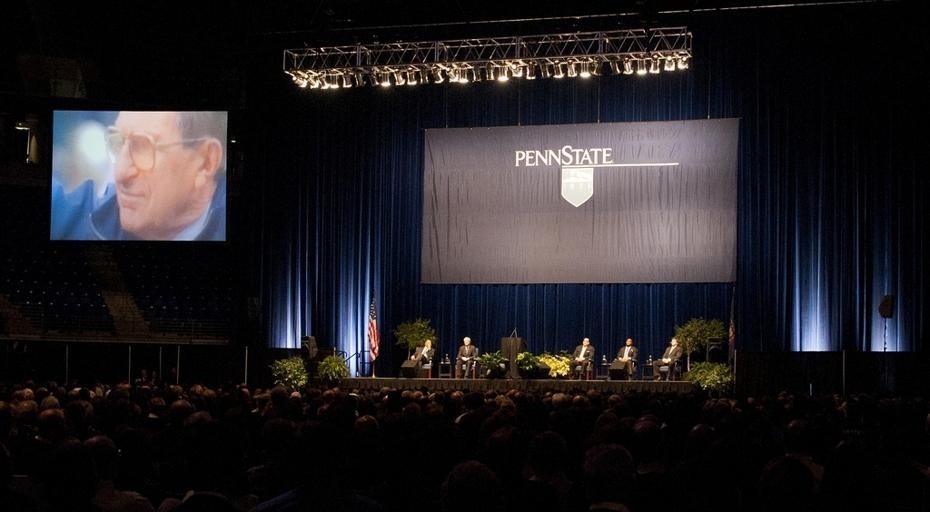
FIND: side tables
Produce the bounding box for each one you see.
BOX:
[436,363,455,378]
[641,364,654,380]
[594,362,611,380]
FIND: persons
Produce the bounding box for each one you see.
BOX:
[657,337,683,381]
[617,337,638,381]
[1,375,929,512]
[410,338,435,365]
[568,337,594,380]
[49,111,227,242]
[454,336,476,380]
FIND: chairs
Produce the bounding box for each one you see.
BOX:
[573,346,595,380]
[416,347,434,378]
[454,348,479,379]
[657,354,681,381]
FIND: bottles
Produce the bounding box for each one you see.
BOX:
[356,370,359,377]
[444,354,450,364]
[602,355,606,365]
[648,354,653,365]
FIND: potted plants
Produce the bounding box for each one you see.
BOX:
[317,355,348,389]
[515,349,575,380]
[474,349,509,378]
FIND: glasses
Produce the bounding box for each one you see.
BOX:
[105,127,207,173]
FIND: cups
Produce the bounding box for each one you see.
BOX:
[646,359,649,365]
[440,358,445,365]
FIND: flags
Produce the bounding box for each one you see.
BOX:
[726,293,737,365]
[366,297,382,362]
